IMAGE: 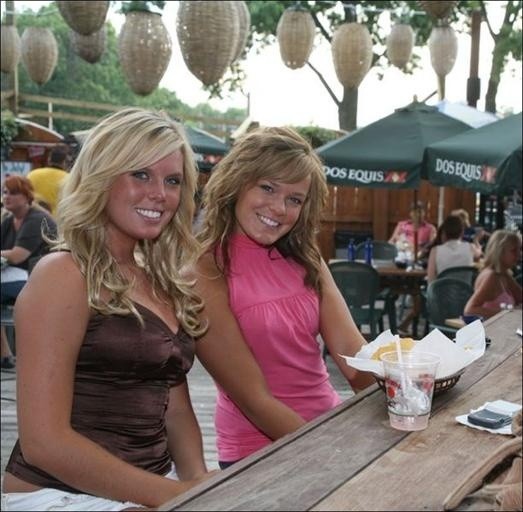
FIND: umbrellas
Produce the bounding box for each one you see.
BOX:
[424,110,523,195]
[311,101,477,266]
[70,119,229,174]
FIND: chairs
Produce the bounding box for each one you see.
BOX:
[323,261,385,355]
[426,279,473,335]
[353,240,400,336]
[422,267,478,338]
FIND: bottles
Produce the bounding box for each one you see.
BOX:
[347,239,357,262]
[364,238,372,265]
[395,249,408,269]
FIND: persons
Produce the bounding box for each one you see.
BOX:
[0,106,219,511]
[1,145,74,370]
[182,125,381,472]
[386,204,523,336]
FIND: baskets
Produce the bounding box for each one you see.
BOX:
[371,370,462,401]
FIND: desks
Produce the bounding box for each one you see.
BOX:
[374,265,427,337]
[0,267,28,328]
[157,305,522,512]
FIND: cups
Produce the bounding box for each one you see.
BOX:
[379,352,441,430]
